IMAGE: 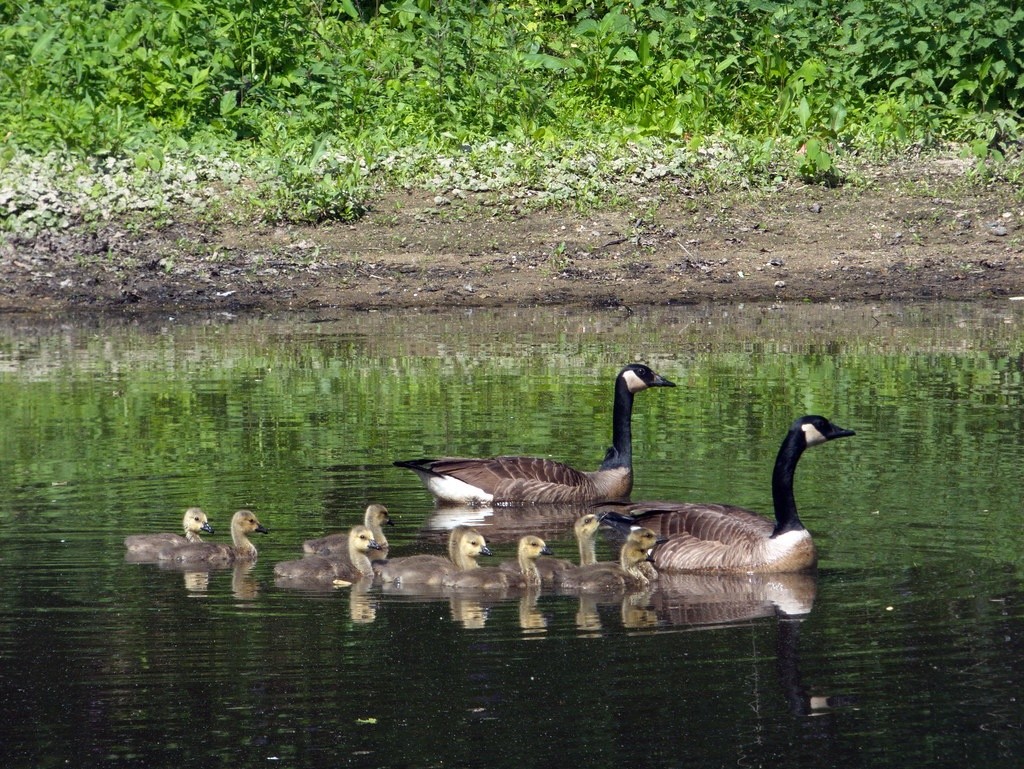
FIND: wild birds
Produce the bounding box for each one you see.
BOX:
[618,573,842,720]
[553,528,670,592]
[303,504,394,560]
[124,508,215,554]
[383,581,506,629]
[159,556,262,599]
[371,519,492,583]
[440,535,554,589]
[500,511,609,580]
[423,502,635,544]
[274,574,392,624]
[393,363,676,507]
[273,524,382,580]
[158,510,269,561]
[587,413,855,573]
